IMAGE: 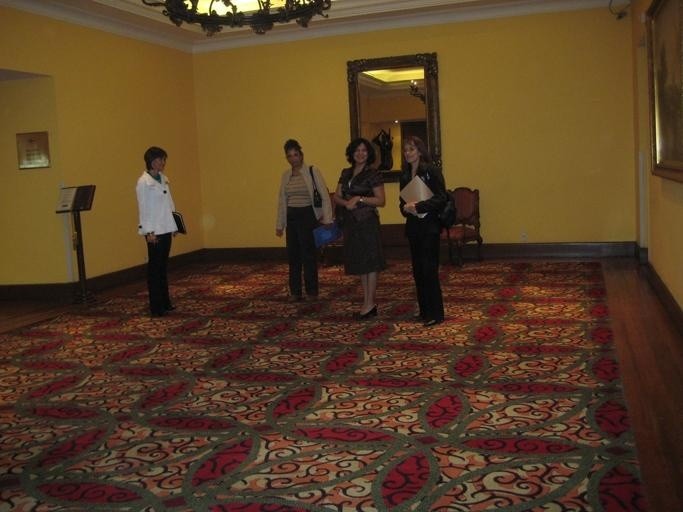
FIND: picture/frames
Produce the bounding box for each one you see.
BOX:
[644,0,681,183]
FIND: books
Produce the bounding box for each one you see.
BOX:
[172,212,187,234]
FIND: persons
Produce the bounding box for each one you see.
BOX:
[398,136,447,327]
[275,139,333,301]
[136,147,178,316]
[333,138,385,321]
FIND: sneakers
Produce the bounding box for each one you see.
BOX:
[307,295,320,301]
[287,296,300,302]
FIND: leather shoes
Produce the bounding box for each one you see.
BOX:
[414,312,442,327]
[353,305,377,321]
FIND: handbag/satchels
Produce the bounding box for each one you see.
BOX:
[313,189,323,208]
[335,205,345,226]
[439,191,456,230]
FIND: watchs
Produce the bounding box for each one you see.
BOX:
[359,196,363,208]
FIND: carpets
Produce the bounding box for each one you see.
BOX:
[1,255,645,511]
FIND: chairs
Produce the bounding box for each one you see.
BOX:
[309,191,342,269]
[436,185,483,266]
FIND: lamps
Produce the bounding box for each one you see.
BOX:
[142,0,332,40]
